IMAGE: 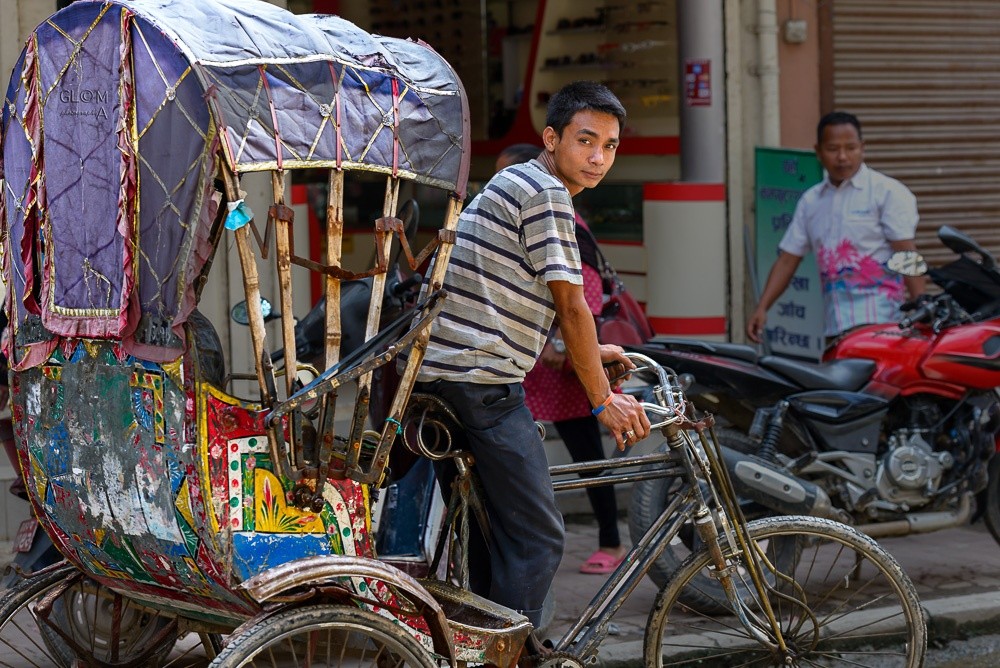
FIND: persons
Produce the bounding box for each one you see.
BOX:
[746,111,925,346]
[394,78,651,668]
[497,145,633,573]
[0,294,22,589]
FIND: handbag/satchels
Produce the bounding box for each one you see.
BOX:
[594,277,655,347]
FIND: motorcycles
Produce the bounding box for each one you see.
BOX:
[618,223,1000,618]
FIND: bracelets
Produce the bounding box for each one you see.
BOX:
[587,391,616,416]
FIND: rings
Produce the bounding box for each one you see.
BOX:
[625,429,635,438]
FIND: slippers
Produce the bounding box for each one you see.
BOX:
[579,549,627,574]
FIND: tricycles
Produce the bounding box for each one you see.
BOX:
[0,1,933,668]
